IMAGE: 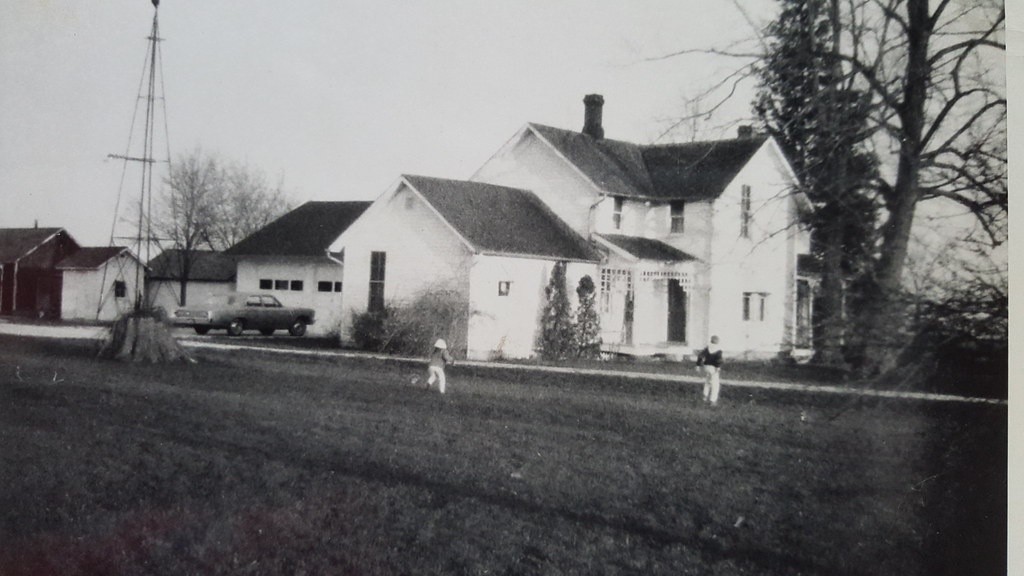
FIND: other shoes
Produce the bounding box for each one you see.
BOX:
[710,401,718,409]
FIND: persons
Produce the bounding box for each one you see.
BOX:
[695,336,724,406]
[425,339,452,393]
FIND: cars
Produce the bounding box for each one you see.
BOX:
[169,292,317,337]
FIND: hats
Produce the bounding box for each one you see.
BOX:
[434,338,448,349]
